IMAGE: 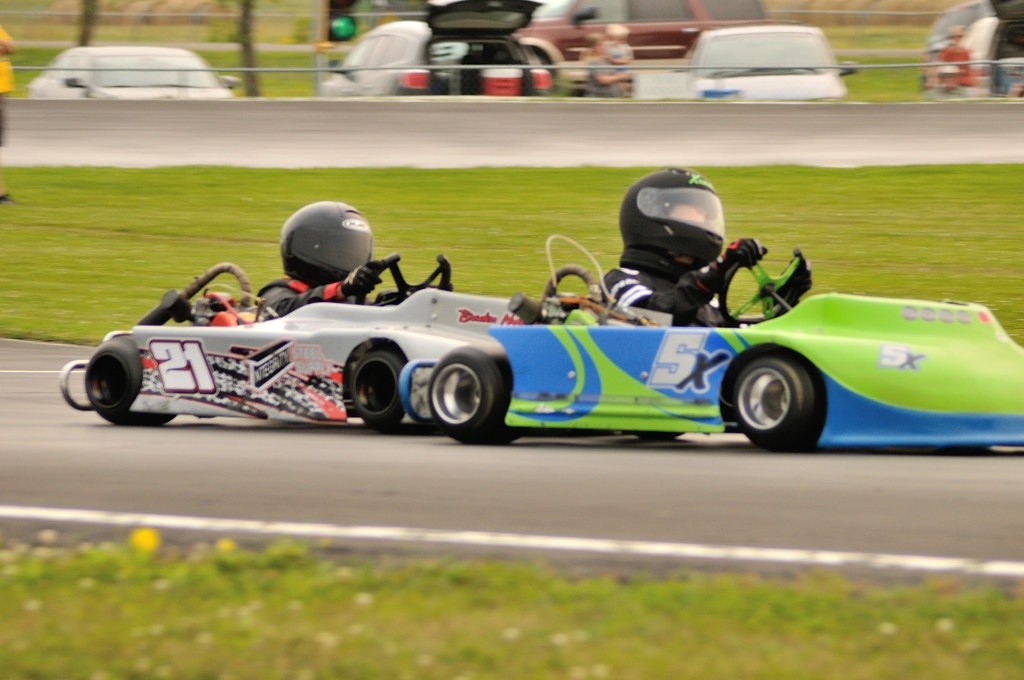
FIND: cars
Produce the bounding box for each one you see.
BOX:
[29,47,245,101]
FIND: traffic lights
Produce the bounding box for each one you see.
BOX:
[324,2,355,43]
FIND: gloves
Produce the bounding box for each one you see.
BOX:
[712,236,768,269]
[342,264,382,292]
[772,248,812,310]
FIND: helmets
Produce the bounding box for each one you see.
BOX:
[618,166,725,278]
[279,200,373,285]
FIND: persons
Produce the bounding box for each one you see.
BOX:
[256,200,382,323]
[922,24,988,100]
[599,167,812,326]
[585,23,634,98]
[0,26,17,204]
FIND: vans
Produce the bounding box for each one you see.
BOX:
[679,21,859,100]
[319,0,558,98]
[518,0,764,59]
